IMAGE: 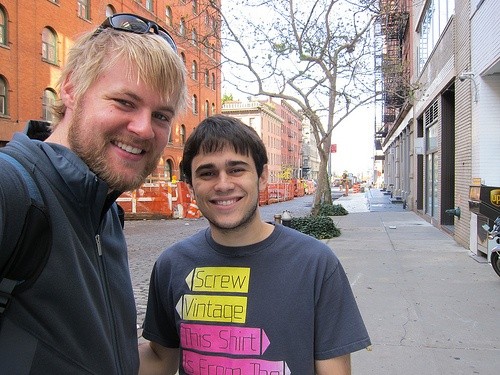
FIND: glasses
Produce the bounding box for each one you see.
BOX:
[87,13,178,54]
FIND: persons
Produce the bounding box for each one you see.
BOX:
[0,12,191,375]
[136,113,372,374]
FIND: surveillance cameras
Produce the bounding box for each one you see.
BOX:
[459,71,475,82]
